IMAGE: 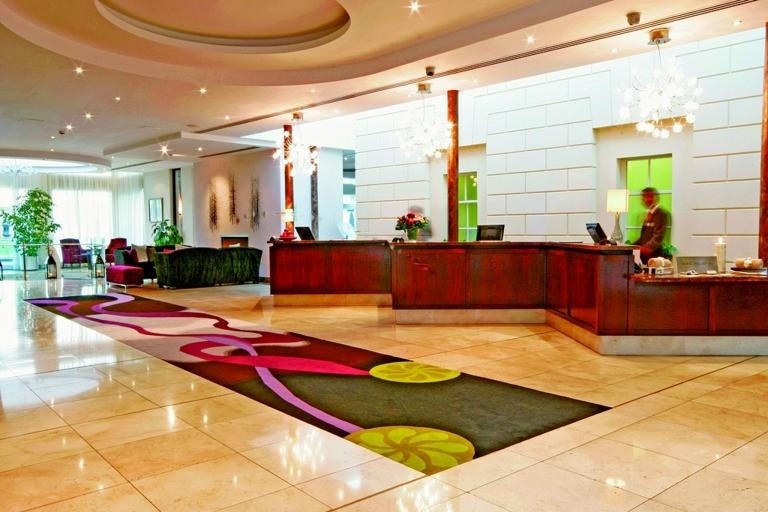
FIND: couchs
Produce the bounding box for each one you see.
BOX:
[151,247,263,289]
[113,244,192,284]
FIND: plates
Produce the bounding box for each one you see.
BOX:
[731,268,767,273]
[278,237,297,241]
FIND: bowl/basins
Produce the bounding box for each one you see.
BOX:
[653,268,674,274]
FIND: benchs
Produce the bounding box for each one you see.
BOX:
[105,266,144,289]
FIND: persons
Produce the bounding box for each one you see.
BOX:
[629,186,667,274]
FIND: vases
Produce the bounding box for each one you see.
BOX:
[407,228,419,241]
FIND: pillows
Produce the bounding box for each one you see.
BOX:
[124,243,191,262]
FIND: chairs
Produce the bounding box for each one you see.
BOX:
[60,238,92,268]
[105,238,127,266]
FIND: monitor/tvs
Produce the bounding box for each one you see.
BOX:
[586,223,607,243]
[477,225,504,241]
[295,227,315,240]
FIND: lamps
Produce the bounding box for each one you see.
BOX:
[284,209,294,229]
[404,83,453,159]
[607,188,629,242]
[272,113,318,178]
[620,28,704,138]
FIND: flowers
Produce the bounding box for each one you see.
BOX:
[395,213,430,231]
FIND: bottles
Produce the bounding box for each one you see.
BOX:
[0,260,4,281]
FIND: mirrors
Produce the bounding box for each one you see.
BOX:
[148,197,163,222]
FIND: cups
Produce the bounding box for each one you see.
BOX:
[715,242,727,274]
